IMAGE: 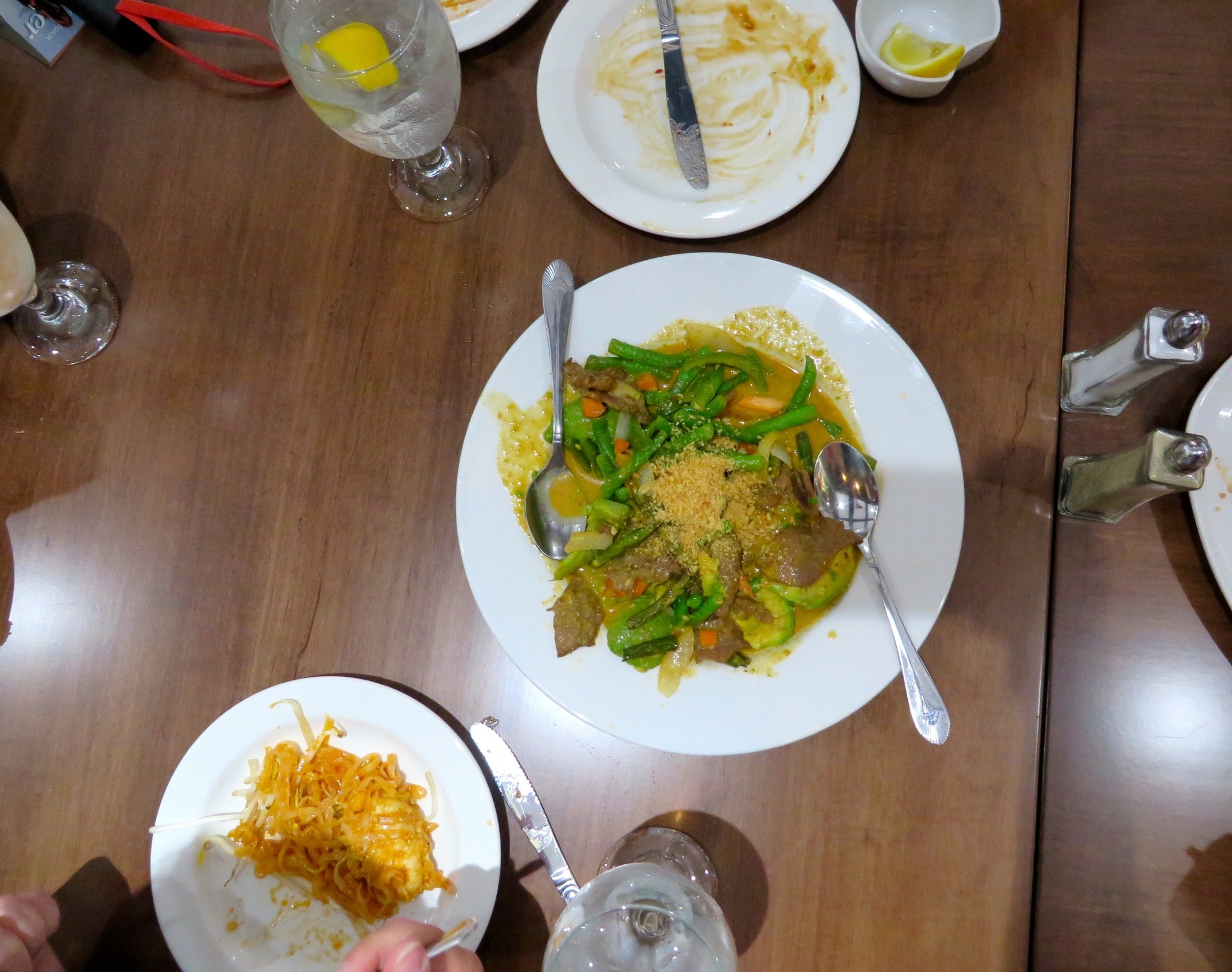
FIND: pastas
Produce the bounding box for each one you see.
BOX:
[196,699,456,937]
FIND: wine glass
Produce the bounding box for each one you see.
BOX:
[541,824,737,972]
[0,198,118,364]
[270,0,492,224]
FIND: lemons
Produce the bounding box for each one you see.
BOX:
[299,21,399,126]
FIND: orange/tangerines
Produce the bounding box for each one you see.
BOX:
[880,26,964,79]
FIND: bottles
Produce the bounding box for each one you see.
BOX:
[1055,428,1211,525]
[1061,301,1208,418]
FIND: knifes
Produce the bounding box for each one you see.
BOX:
[469,721,580,908]
[653,0,711,192]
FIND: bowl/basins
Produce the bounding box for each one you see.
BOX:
[852,0,1002,97]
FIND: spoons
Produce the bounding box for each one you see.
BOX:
[813,440,951,745]
[525,258,586,561]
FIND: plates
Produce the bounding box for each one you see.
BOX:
[1186,349,1230,610]
[537,0,865,239]
[151,673,502,972]
[455,254,968,760]
[385,1,532,59]
